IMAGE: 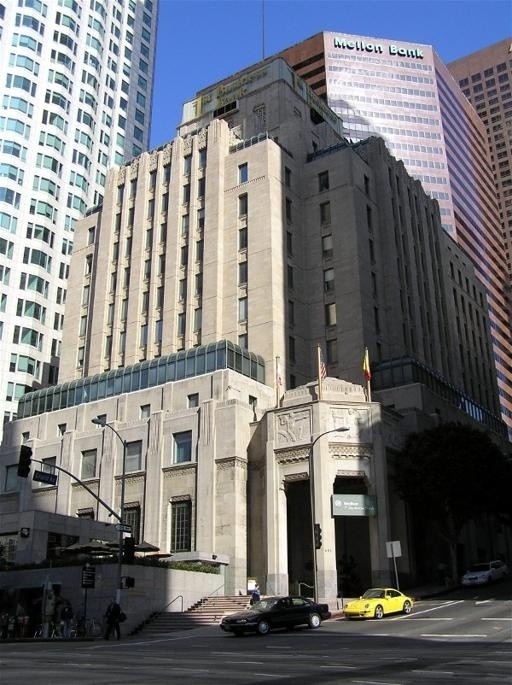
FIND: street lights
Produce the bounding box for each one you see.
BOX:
[92,418,126,641]
[310,426,350,603]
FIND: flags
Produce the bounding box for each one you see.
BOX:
[361,349,373,380]
[319,348,327,381]
[277,358,283,386]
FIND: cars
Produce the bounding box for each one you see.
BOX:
[343,588,416,620]
[220,595,331,636]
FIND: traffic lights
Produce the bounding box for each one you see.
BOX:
[16,444,32,478]
[314,523,322,548]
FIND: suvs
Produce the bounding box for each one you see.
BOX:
[461,560,509,587]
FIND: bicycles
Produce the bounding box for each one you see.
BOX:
[33,623,78,639]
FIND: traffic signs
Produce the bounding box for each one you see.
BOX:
[114,523,133,533]
[32,470,58,485]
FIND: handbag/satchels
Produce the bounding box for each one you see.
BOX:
[119,612,127,622]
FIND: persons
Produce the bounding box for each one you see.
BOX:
[61,599,73,640]
[0,608,9,640]
[102,597,122,641]
[252,583,260,604]
[9,611,23,638]
